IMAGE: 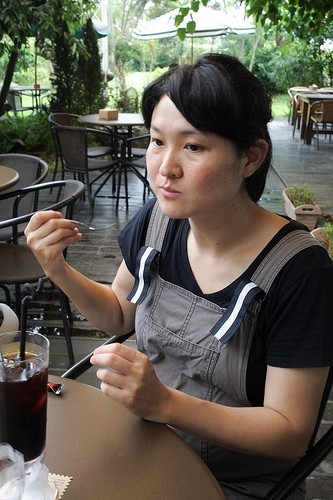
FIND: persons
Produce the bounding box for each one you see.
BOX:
[24,54,333,500]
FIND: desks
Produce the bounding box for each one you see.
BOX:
[298,94,333,146]
[77,114,146,211]
[0,375,228,500]
[21,87,52,117]
[0,165,20,191]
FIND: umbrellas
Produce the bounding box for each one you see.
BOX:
[130,1,255,66]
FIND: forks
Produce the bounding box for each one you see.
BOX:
[62,218,116,231]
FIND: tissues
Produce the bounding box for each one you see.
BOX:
[98,106,118,121]
[309,84,318,90]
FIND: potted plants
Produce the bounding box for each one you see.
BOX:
[310,221,333,263]
[282,182,322,231]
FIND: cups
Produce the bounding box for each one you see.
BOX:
[0,330,51,464]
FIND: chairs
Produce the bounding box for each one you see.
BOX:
[59,327,333,500]
[0,112,151,369]
[5,90,34,118]
[288,86,333,147]
